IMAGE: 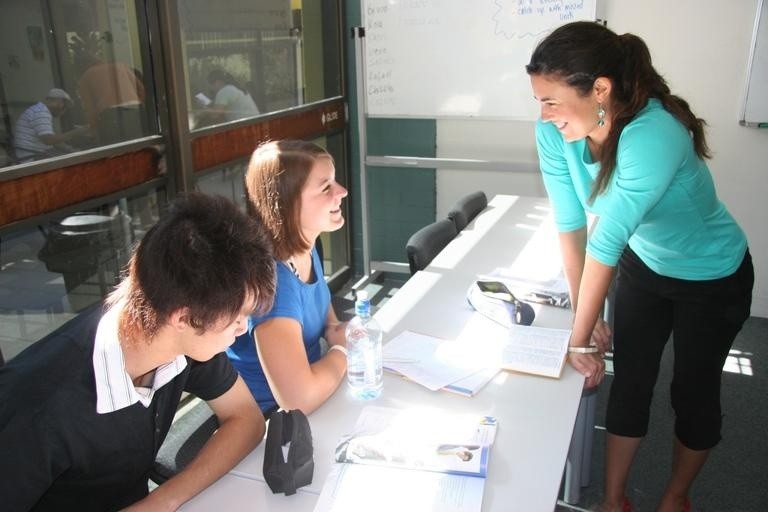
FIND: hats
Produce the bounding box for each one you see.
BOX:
[48,88,75,107]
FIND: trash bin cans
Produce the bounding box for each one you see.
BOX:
[58,214,123,315]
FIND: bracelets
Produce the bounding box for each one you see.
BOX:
[568,346,599,353]
[328,345,347,356]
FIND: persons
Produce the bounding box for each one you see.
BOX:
[225,140,366,420]
[0,196,265,512]
[525,20,754,512]
[13,58,258,179]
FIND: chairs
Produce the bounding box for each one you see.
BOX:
[40,216,120,300]
[0,134,49,164]
[448,193,489,231]
[148,394,220,485]
[406,217,457,274]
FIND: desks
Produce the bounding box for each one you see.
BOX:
[180,194,606,507]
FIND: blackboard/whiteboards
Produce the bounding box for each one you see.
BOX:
[361,0,598,116]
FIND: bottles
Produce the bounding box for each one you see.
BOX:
[346,291,383,401]
